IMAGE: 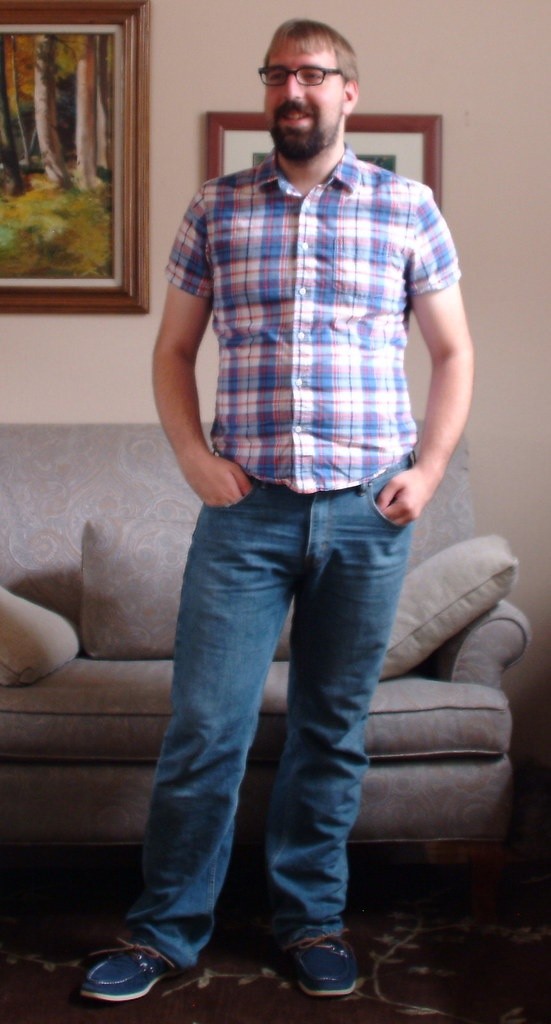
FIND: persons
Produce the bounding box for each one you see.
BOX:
[79,18,473,1002]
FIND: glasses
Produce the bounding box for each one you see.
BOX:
[257,66,343,85]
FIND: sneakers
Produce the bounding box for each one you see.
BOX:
[287,930,356,995]
[81,936,179,1001]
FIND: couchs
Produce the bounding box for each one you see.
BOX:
[0,422,527,924]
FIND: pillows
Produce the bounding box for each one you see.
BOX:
[0,581,79,687]
[379,534,518,676]
[79,514,295,660]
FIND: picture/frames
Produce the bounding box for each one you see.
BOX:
[0,0,151,316]
[207,111,441,216]
